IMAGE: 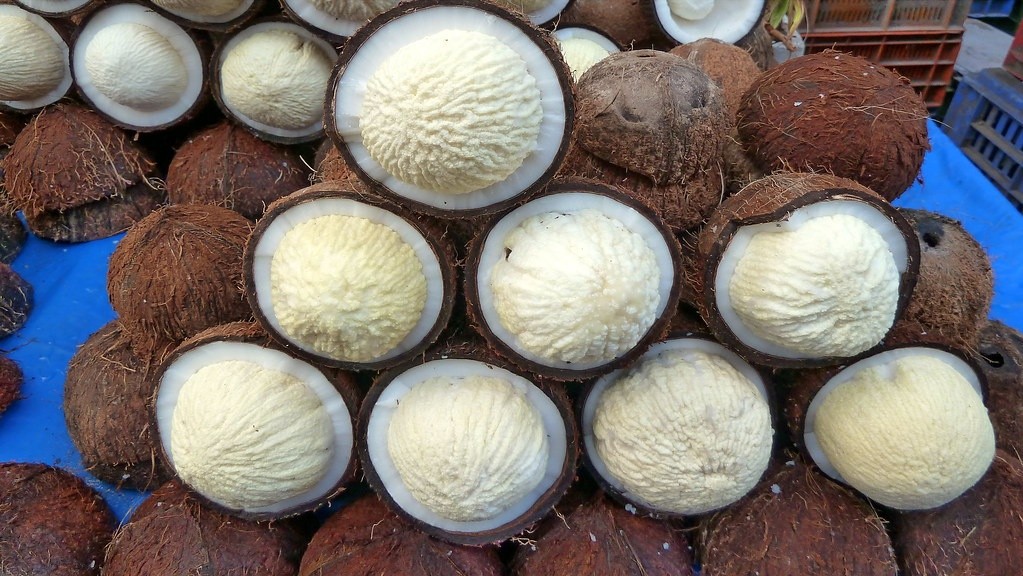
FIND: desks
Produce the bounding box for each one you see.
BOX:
[0,118,1023,536]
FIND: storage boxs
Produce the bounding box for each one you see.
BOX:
[799,31,962,115]
[942,69,1023,216]
[797,0,972,34]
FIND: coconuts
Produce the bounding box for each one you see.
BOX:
[0,0,1023,576]
